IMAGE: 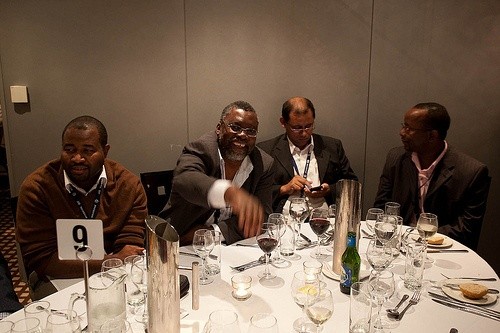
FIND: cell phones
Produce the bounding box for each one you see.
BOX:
[309,186,322,192]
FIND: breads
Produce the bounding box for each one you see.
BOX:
[423,236,444,244]
[458,283,488,299]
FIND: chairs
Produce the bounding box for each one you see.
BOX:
[139,170,176,217]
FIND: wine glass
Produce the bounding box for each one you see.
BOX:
[256,197,339,333]
[193,229,215,285]
[363,202,438,333]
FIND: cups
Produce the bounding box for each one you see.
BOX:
[231,274,252,300]
[347,282,373,333]
[203,230,221,275]
[247,313,279,333]
[202,309,242,333]
[0,250,148,333]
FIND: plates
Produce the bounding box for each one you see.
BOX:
[441,279,497,306]
[427,235,453,248]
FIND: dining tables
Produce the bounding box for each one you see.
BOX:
[0,219,500,333]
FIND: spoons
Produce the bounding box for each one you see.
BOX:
[386,294,409,315]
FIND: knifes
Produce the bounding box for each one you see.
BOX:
[427,291,500,323]
[427,249,469,253]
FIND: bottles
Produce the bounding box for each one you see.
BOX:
[340,231,361,295]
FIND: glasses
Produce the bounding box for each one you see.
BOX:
[400,121,431,134]
[284,121,316,134]
[221,119,258,138]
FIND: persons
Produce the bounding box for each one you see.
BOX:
[14,116,148,301]
[256,97,357,223]
[373,102,489,251]
[160,100,275,245]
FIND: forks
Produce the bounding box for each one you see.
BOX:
[395,291,421,321]
